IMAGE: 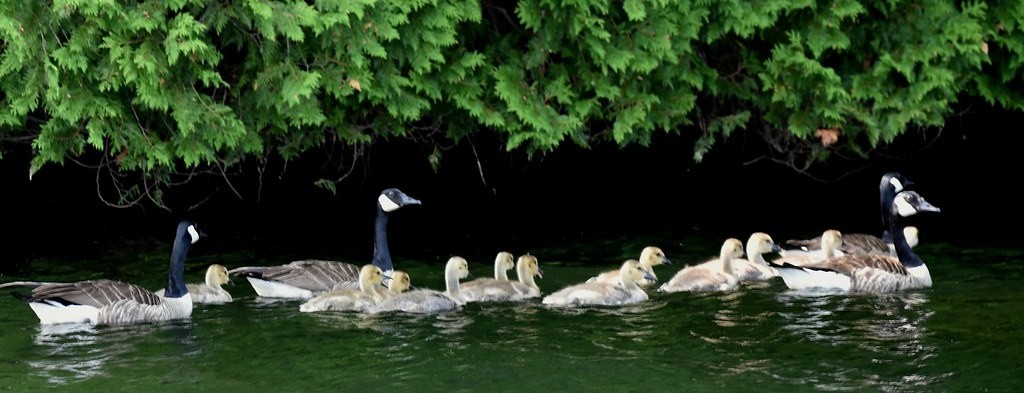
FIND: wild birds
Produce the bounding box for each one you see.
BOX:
[154,265,236,305]
[659,232,782,293]
[230,188,546,315]
[1,221,209,326]
[771,172,942,294]
[543,246,675,308]
